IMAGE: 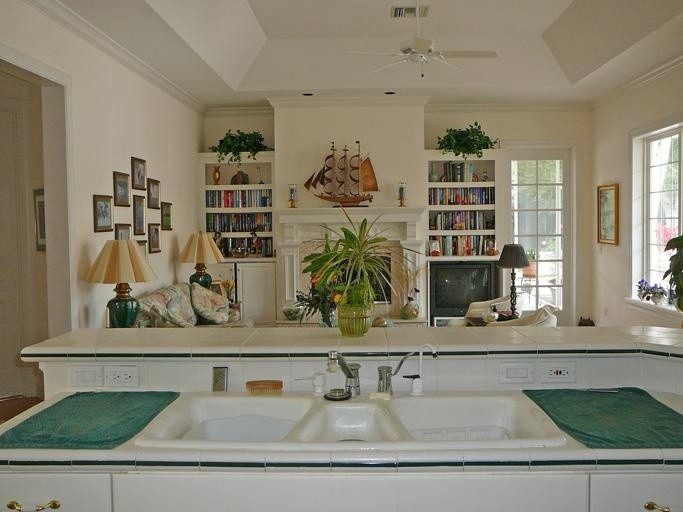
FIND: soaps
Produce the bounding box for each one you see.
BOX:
[368,392,392,401]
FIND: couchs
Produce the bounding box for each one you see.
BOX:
[468,294,510,315]
[486,305,557,327]
[135,282,254,328]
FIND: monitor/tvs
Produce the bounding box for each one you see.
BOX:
[427,263,498,316]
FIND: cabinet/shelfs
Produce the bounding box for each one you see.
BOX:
[202,150,276,255]
[0,471,113,511]
[207,263,276,327]
[426,150,512,260]
[590,472,683,512]
[113,472,588,512]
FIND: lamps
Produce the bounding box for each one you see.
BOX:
[176,229,226,289]
[496,244,530,320]
[86,239,159,328]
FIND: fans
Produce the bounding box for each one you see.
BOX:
[342,0,499,82]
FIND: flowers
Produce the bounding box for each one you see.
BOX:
[296,272,344,320]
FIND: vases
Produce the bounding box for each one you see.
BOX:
[317,309,335,328]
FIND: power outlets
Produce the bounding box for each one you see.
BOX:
[104,365,140,387]
[537,361,576,383]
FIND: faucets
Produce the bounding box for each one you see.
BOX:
[379,343,437,391]
[325,351,360,396]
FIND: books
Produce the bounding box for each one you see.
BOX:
[430,187,495,205]
[207,213,272,232]
[206,189,272,208]
[437,211,487,230]
[218,237,272,258]
[429,236,495,255]
[444,161,475,182]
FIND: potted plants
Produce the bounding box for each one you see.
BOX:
[301,204,422,337]
[394,264,428,319]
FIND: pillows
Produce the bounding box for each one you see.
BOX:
[191,281,230,323]
[151,305,195,328]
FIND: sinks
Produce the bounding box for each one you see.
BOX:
[133,392,320,450]
[291,393,408,452]
[387,388,567,450]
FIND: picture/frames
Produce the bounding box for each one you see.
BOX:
[93,195,114,233]
[148,222,163,253]
[33,188,45,252]
[113,170,131,207]
[132,194,147,235]
[210,282,224,297]
[146,178,161,209]
[115,223,132,240]
[131,157,147,190]
[137,240,148,261]
[597,183,620,245]
[160,201,173,230]
